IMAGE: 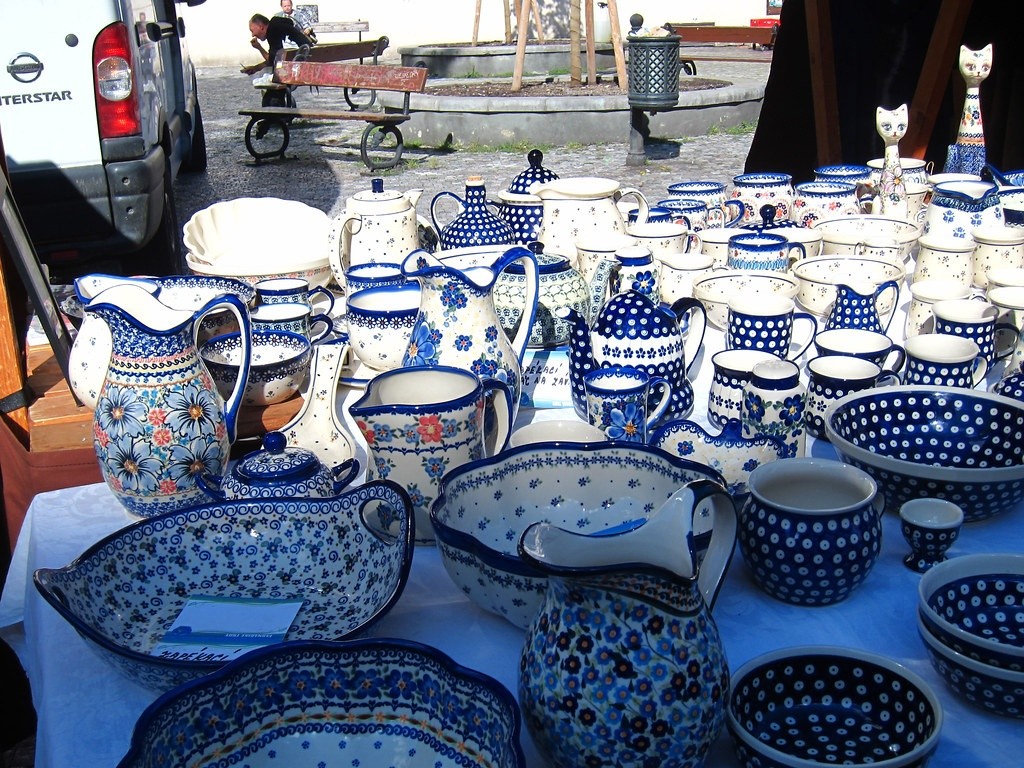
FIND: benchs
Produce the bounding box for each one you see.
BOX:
[270,34,392,126]
[666,23,780,78]
[240,58,428,181]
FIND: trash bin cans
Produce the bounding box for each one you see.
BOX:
[626,33,684,108]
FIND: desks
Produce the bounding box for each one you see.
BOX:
[0,270,1024,766]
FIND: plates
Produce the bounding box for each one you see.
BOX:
[110,633,525,768]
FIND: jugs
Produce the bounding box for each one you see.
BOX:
[68,272,164,412]
[355,363,514,545]
[612,248,660,296]
[516,479,740,767]
[581,367,672,447]
[400,243,542,447]
[88,295,254,523]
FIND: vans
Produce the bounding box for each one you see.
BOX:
[1,0,208,275]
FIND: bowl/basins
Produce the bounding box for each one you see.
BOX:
[181,191,341,306]
[32,480,420,698]
[144,275,258,344]
[345,282,425,371]
[426,440,728,629]
[915,550,1023,717]
[724,643,943,768]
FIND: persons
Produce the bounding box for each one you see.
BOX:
[240,14,313,107]
[274,0,312,36]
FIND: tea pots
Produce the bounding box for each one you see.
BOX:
[488,236,623,350]
[330,153,648,295]
[557,285,708,427]
[194,432,361,512]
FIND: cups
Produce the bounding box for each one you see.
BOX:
[575,241,618,289]
[247,306,333,346]
[510,416,609,452]
[344,262,406,299]
[255,277,336,322]
[643,142,1023,605]
